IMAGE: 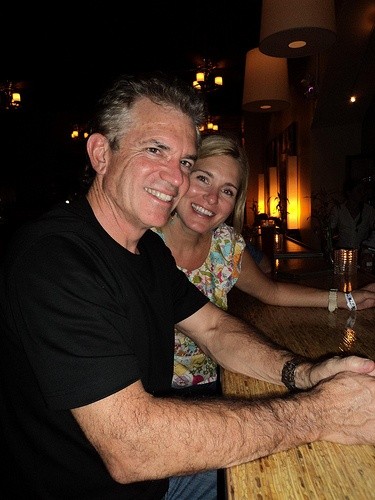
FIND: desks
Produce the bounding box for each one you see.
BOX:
[219,253,375,496]
[243,234,316,256]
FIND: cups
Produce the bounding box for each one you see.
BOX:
[336,249,357,271]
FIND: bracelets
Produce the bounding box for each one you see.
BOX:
[328,289,358,312]
[281,357,306,393]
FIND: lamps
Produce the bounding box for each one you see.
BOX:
[258,0,339,58]
[243,48,290,114]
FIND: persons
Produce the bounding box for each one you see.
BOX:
[321,178,375,273]
[0,73,375,500]
[149,135,375,398]
